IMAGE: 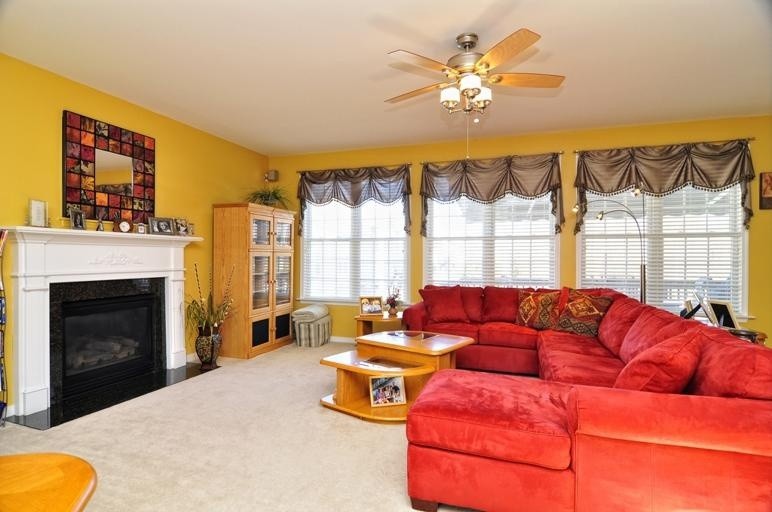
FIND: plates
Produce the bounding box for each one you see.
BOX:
[49,217,70,228]
[119,222,130,232]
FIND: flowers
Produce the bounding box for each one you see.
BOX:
[385,286,402,305]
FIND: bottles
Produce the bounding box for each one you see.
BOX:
[138,223,144,233]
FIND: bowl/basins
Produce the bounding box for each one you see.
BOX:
[102,221,114,232]
[85,220,99,230]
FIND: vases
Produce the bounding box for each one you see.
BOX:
[388,304,398,315]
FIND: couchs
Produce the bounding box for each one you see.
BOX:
[404,276,772,512]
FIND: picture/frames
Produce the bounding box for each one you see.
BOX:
[62,110,155,227]
[28,198,48,228]
[369,376,406,407]
[113,217,192,236]
[69,208,86,229]
[359,296,384,318]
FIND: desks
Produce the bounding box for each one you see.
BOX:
[0,453,97,511]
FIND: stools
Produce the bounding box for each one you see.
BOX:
[295,314,332,347]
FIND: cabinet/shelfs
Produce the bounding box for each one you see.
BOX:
[212,201,297,359]
[354,311,403,337]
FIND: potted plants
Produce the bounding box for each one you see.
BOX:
[180,262,235,370]
[245,184,292,209]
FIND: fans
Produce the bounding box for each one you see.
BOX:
[384,28,565,104]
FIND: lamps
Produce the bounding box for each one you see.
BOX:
[440,76,492,113]
[572,199,646,306]
